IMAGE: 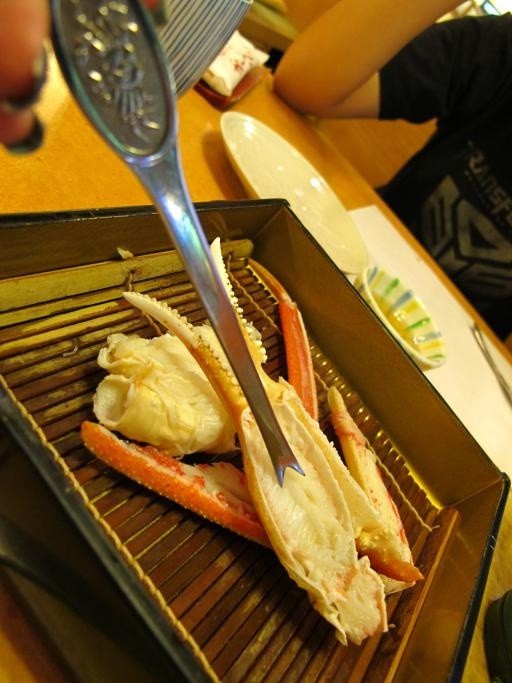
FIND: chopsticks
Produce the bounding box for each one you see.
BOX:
[470,321,512,409]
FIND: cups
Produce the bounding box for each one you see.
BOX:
[154,1,253,101]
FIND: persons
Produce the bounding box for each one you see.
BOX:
[1,0,50,147]
[275,1,512,356]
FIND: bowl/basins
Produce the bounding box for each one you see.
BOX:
[353,263,446,370]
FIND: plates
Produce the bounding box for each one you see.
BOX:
[216,109,373,276]
[195,68,262,107]
